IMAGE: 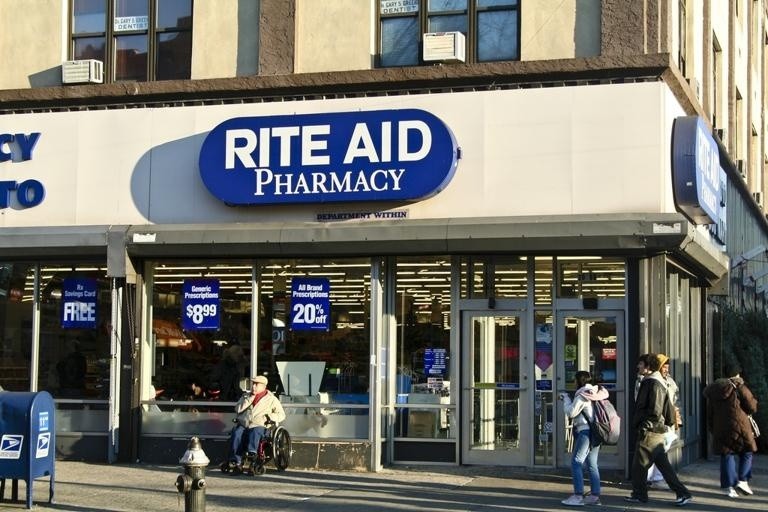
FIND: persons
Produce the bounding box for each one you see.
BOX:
[560,370,603,506]
[624,353,693,506]
[229,376,286,477]
[703,361,759,498]
[227,345,251,401]
[47,351,70,389]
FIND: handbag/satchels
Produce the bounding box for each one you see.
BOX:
[747,413,760,439]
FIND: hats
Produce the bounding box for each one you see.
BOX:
[250,376,268,384]
[725,363,743,376]
[656,353,669,370]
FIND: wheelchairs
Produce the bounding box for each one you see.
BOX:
[220,414,291,476]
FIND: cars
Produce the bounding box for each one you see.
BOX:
[318,372,369,415]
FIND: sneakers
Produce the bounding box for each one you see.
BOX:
[561,480,754,506]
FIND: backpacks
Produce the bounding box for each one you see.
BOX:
[577,389,620,447]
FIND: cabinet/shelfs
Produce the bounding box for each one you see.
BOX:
[0,348,30,391]
